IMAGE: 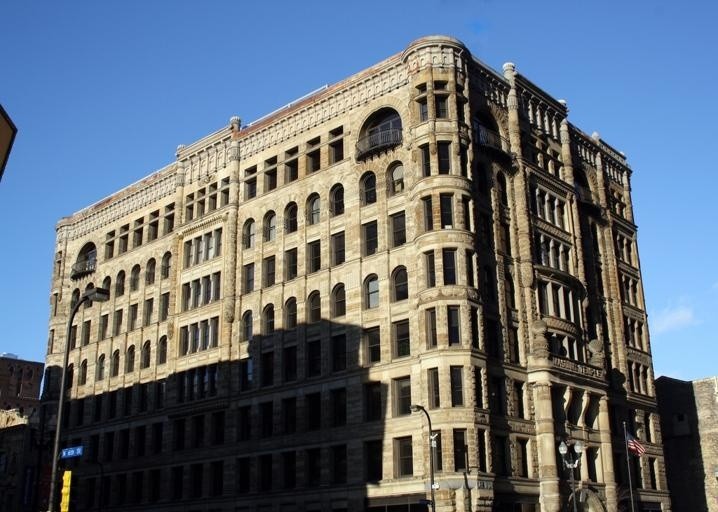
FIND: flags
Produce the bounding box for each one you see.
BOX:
[624,432,646,456]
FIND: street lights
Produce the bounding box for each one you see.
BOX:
[45,287,110,511]
[409,405,436,511]
[558,438,588,512]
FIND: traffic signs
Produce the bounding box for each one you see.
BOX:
[60,446,82,457]
